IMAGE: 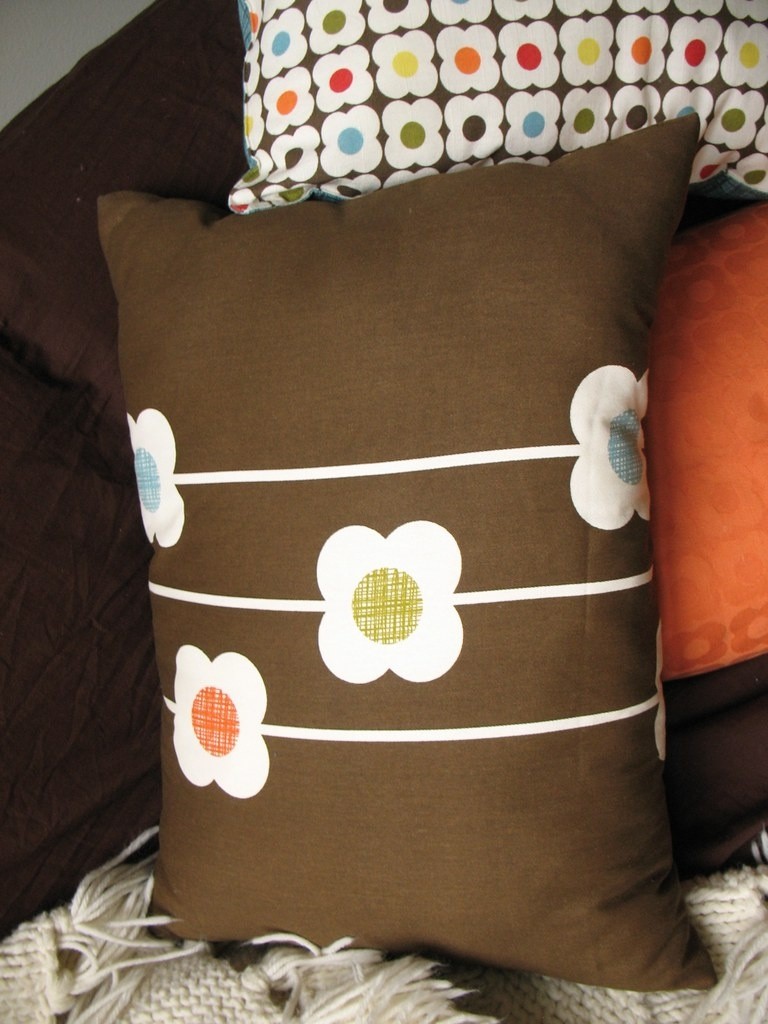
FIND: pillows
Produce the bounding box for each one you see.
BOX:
[646,196,768,684]
[224,0,768,217]
[96,113,720,994]
